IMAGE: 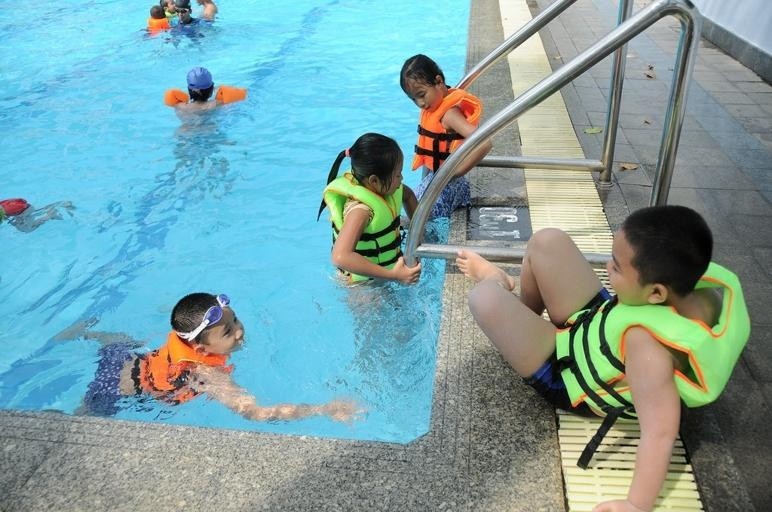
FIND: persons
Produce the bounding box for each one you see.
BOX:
[43,291,368,429]
[196,0,219,21]
[159,0,177,19]
[455,204,751,511]
[398,55,495,227]
[148,6,171,33]
[171,66,223,140]
[314,133,421,373]
[170,1,212,43]
[1,196,80,233]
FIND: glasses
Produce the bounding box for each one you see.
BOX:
[174,8,187,12]
[185,293,231,342]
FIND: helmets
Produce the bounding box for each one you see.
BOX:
[187,66,213,89]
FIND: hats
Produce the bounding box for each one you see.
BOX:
[175,0,191,7]
